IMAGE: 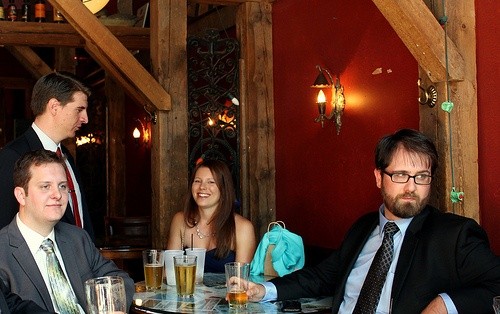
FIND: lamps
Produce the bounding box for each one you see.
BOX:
[311,65,345,136]
[133,118,149,149]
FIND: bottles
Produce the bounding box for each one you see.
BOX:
[21,0,31,22]
[53,7,66,24]
[7,0,18,22]
[0,0,4,21]
[33,0,46,23]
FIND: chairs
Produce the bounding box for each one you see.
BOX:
[103,216,152,247]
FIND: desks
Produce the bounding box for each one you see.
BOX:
[99,248,150,259]
[130,273,332,314]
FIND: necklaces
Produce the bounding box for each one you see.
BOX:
[193,212,215,238]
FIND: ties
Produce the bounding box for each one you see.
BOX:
[56,147,82,229]
[353,222,401,314]
[40,238,81,314]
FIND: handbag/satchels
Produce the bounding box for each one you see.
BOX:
[250,220,305,284]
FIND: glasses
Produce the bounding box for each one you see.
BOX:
[384,170,434,185]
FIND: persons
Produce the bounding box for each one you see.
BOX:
[167,159,257,278]
[0,149,135,313]
[0,70,94,242]
[225,131,500,314]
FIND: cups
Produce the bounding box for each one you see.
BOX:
[142,249,165,292]
[173,254,197,299]
[185,247,207,285]
[164,249,184,286]
[224,262,250,311]
[84,275,127,314]
